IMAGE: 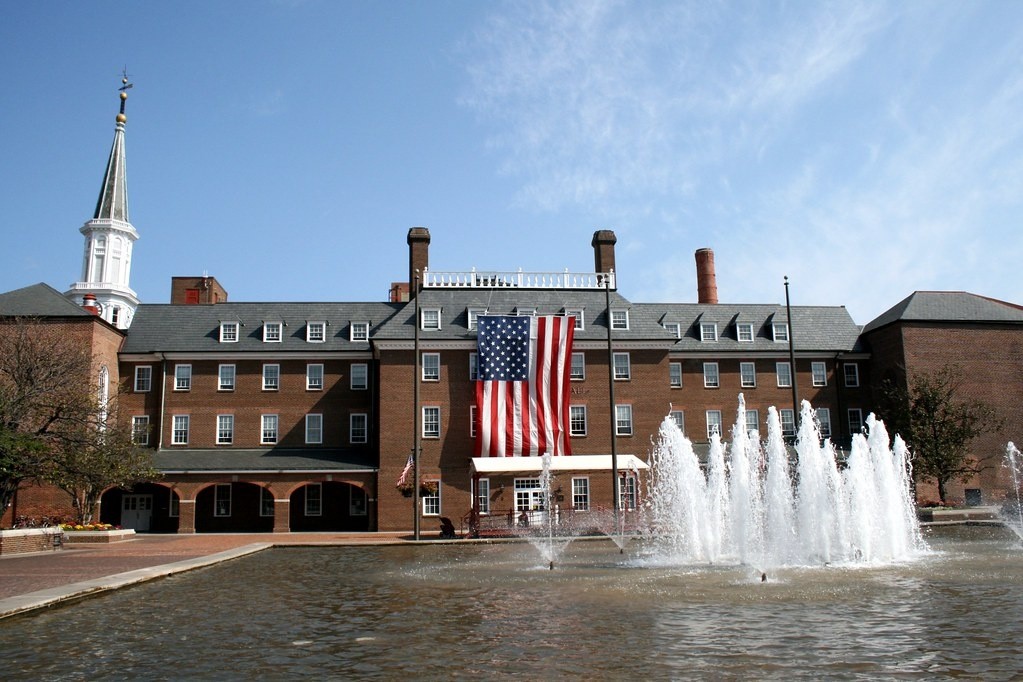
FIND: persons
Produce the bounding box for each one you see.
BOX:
[518,511,528,527]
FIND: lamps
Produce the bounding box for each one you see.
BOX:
[558,484,563,492]
[500,483,505,493]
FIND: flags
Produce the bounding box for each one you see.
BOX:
[396,454,414,487]
[473,314,576,458]
